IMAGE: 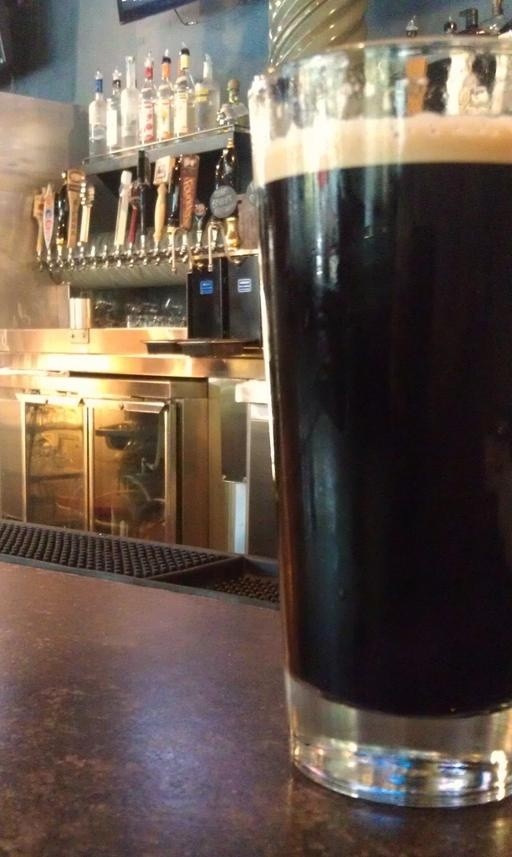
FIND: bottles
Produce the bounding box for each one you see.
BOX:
[87,0,512,166]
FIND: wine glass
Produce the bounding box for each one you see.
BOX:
[95,289,188,329]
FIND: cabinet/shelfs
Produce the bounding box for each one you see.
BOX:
[39,123,262,358]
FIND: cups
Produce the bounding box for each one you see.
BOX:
[241,35,511,812]
